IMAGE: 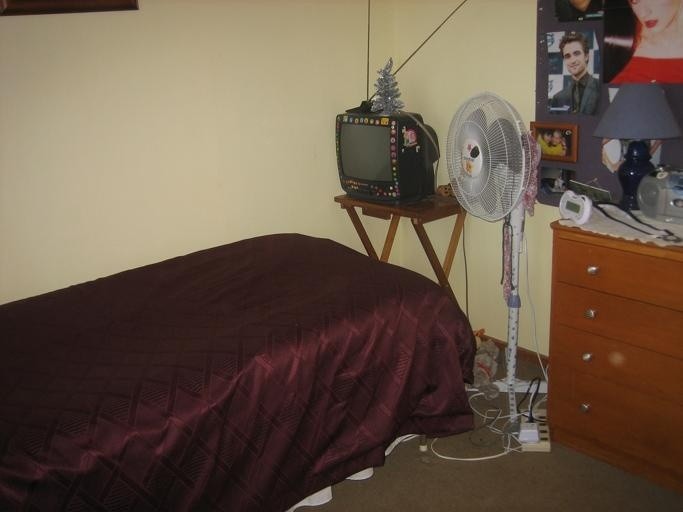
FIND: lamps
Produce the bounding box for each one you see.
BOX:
[591,83,682,212]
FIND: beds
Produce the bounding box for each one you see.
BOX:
[0,232,477,511]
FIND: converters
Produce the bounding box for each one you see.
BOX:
[519,423,540,443]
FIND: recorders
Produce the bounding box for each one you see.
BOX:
[636,165,683,225]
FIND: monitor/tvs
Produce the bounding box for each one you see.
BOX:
[336,109,439,205]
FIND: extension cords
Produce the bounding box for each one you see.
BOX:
[521,409,551,452]
[492,377,548,393]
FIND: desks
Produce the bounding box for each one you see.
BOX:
[334,189,476,327]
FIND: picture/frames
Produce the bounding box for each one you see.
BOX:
[0,0,139,16]
[529,122,577,164]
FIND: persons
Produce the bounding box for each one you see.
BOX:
[537,129,567,158]
[610,0,683,86]
[557,1,606,22]
[552,32,599,113]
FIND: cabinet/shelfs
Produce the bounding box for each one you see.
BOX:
[547,218,683,499]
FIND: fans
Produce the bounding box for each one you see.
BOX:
[444,94,541,434]
